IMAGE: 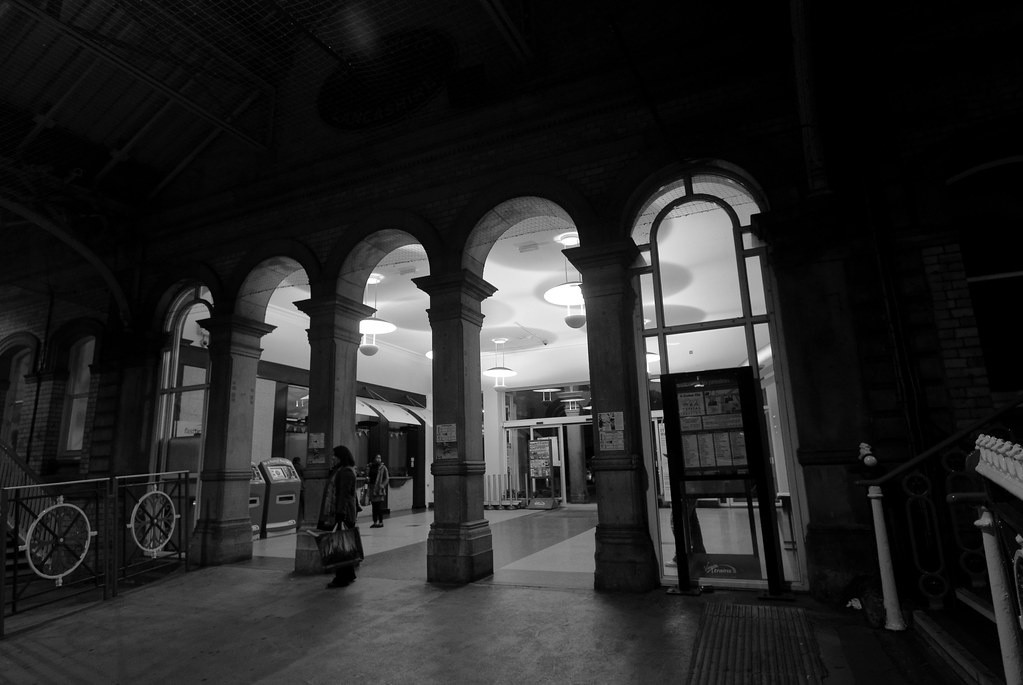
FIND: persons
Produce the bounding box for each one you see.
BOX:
[367,455,389,528]
[318,446,362,588]
[293,457,305,519]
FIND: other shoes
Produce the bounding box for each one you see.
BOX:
[327,577,348,587]
[376,524,383,528]
[345,573,356,581]
[370,524,377,528]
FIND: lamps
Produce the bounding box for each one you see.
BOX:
[483,338,517,392]
[358,272,397,356]
[536,388,584,415]
[644,319,660,364]
[543,232,586,329]
